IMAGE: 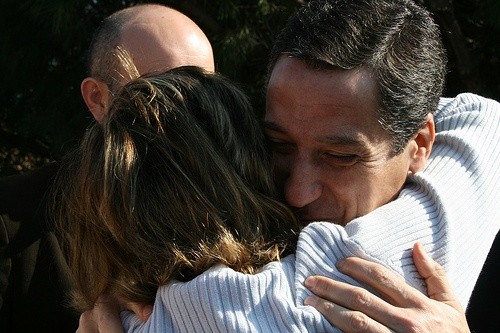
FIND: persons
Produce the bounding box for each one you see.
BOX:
[47,66,500,333]
[76,0,498,332]
[1,4,216,333]
